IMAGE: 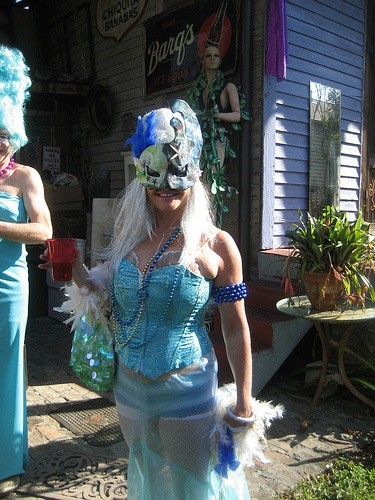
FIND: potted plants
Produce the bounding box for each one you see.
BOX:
[262,202,375,319]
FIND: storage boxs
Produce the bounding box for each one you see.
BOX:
[45,185,84,211]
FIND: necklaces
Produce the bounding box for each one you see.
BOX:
[110,228,183,347]
[0,158,14,176]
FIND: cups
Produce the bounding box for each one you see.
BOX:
[45,238,76,281]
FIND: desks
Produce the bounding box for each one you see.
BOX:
[276,296,375,434]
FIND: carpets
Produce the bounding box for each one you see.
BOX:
[48,398,125,446]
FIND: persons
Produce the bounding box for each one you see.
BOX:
[0,46,53,493]
[187,46,240,222]
[36,107,258,500]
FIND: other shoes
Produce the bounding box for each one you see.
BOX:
[0,475,20,495]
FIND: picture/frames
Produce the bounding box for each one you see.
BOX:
[141,0,241,101]
[60,2,96,81]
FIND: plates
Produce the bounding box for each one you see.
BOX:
[88,85,112,136]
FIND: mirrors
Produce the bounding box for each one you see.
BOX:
[309,81,341,216]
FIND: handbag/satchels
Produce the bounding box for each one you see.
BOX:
[68,301,114,394]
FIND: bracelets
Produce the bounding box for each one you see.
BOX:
[226,406,256,423]
